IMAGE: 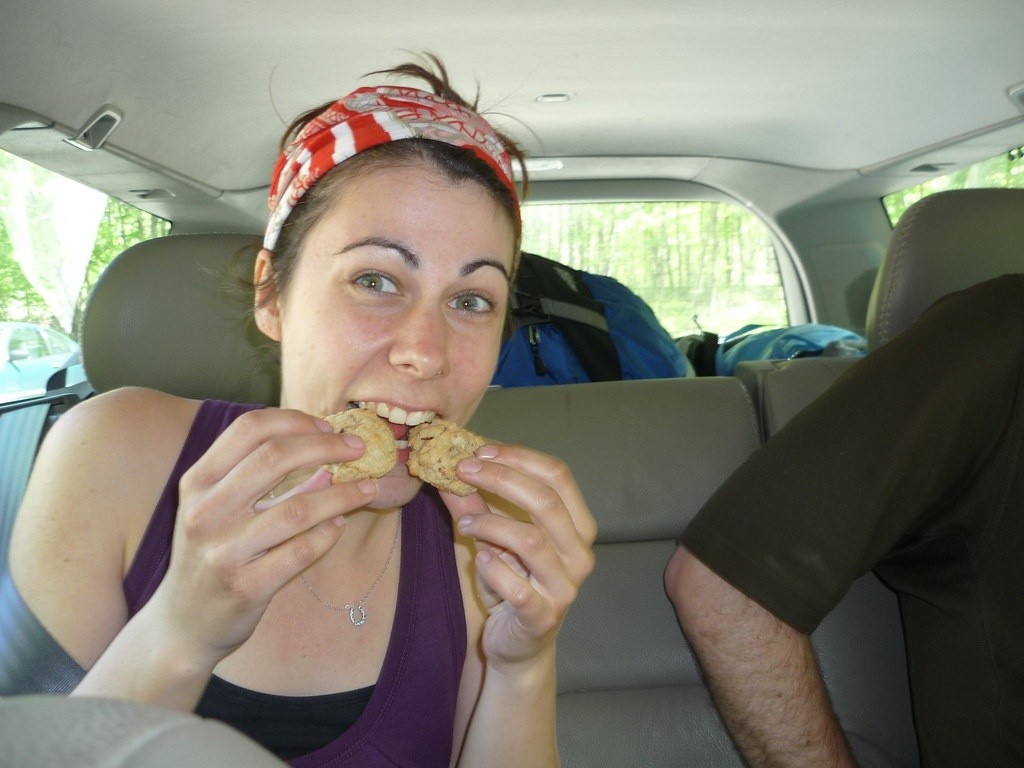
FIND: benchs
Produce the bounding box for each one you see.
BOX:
[82,187,1024,768]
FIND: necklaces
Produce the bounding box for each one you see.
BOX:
[269,489,401,627]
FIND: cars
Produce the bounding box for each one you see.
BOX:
[0,322,83,395]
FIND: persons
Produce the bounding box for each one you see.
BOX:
[0,51,597,768]
[664,271,1024,768]
